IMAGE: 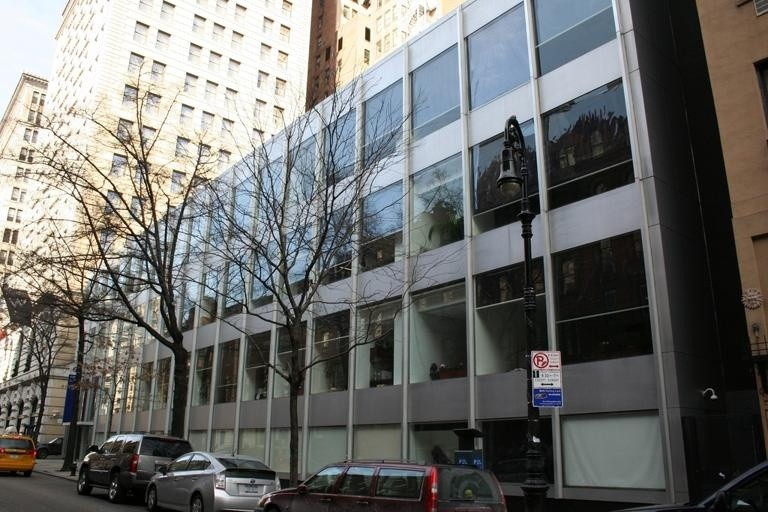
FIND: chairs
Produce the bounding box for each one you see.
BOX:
[344,474,421,499]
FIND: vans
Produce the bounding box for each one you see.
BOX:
[75,433,194,503]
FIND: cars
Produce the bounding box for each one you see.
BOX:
[0,433,38,478]
[37,436,64,459]
[144,451,281,512]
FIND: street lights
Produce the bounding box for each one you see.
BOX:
[495,113,552,512]
